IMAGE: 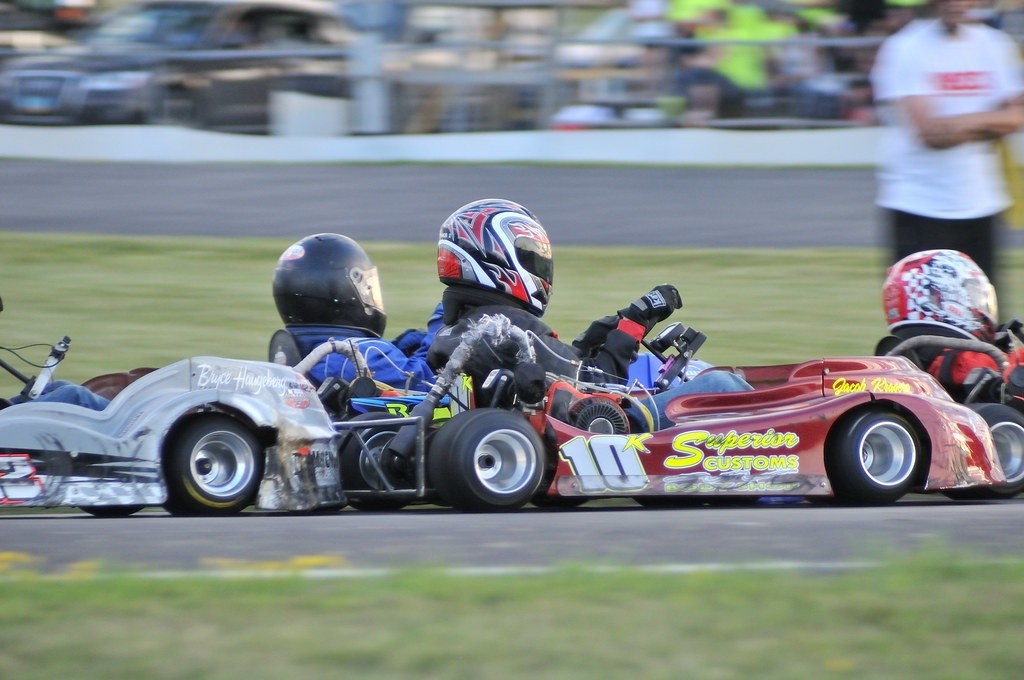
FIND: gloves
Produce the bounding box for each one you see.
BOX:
[618,284,683,341]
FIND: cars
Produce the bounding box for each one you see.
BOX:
[506,0,1024,129]
[0,1,410,134]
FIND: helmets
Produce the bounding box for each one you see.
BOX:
[273,232,387,339]
[438,198,554,317]
[883,248,998,343]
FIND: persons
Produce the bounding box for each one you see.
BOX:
[271,198,755,433]
[876,246,1024,411]
[864,0,1022,312]
[552,0,926,131]
